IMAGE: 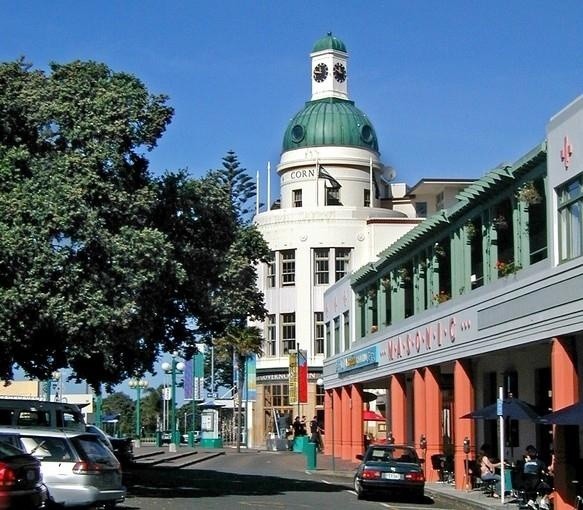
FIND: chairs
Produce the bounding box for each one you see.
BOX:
[431,453,553,510]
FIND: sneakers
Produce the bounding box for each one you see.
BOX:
[539,502,549,510]
[528,501,537,510]
[494,493,499,498]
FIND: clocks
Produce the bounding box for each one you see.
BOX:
[312,62,347,83]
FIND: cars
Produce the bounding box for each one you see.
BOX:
[349,445,426,503]
[0,394,134,510]
[182,430,201,443]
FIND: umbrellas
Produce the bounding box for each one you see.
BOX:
[532,401,583,427]
[458,392,550,456]
[362,410,386,422]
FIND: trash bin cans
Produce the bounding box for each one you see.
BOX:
[304,443,316,470]
[172,432,179,445]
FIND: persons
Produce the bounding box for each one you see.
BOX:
[476,440,583,510]
[286,414,324,452]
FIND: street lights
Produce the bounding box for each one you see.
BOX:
[160,350,186,454]
[128,379,148,439]
[37,367,61,402]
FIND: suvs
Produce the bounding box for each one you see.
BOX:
[159,429,183,446]
